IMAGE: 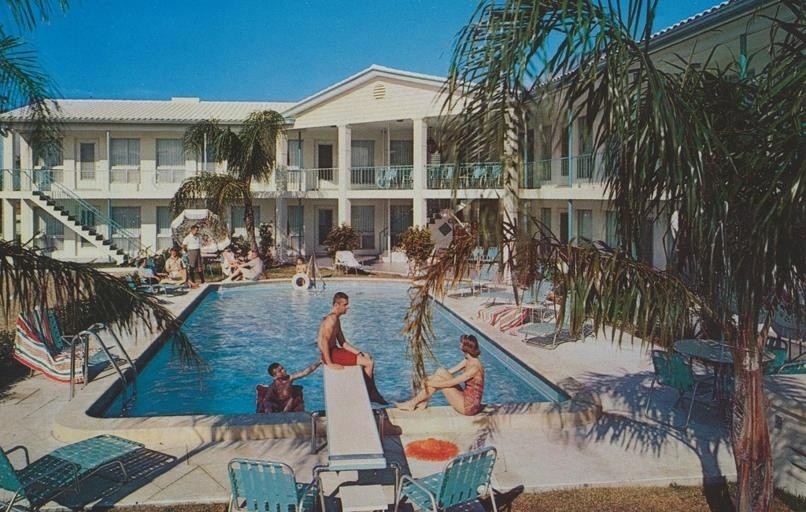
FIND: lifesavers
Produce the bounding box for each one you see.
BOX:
[291,273,310,291]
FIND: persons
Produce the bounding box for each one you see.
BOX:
[137,258,160,284]
[317,291,389,406]
[222,247,243,273]
[220,250,264,282]
[394,333,485,416]
[182,225,206,283]
[164,247,187,281]
[295,257,307,285]
[259,359,325,414]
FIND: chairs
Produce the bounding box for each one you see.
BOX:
[429,167,440,189]
[644,350,724,429]
[228,458,326,512]
[776,352,806,374]
[0,433,144,512]
[334,250,374,276]
[758,333,788,368]
[395,446,499,512]
[125,259,189,296]
[440,166,458,189]
[401,170,413,190]
[470,166,488,186]
[490,164,503,187]
[471,263,501,296]
[517,290,592,346]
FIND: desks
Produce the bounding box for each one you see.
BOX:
[672,338,775,399]
